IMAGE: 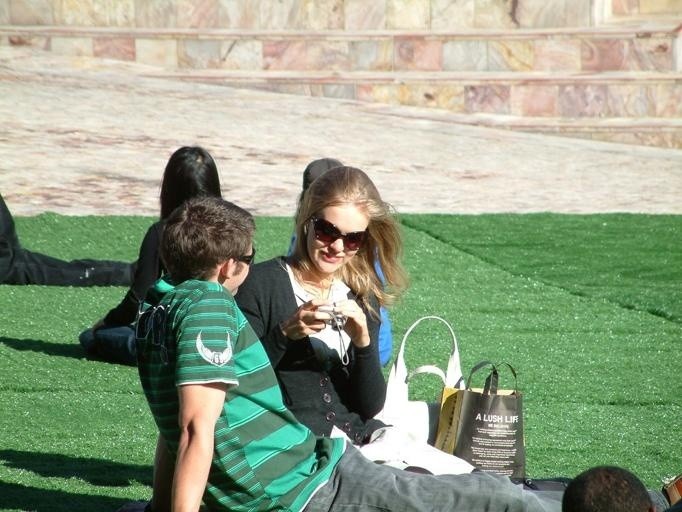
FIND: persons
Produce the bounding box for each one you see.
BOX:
[561,464,656,512]
[287,157,395,369]
[0,194,140,287]
[133,194,681,512]
[233,165,481,475]
[79,146,223,368]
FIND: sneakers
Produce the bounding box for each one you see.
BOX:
[663,475,682,512]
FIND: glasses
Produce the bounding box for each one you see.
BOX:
[306,217,369,251]
[236,248,255,266]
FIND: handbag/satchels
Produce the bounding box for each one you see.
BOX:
[373,316,466,446]
[434,361,526,485]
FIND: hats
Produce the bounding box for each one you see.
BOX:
[303,158,344,190]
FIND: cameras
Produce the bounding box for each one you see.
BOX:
[318,301,347,331]
